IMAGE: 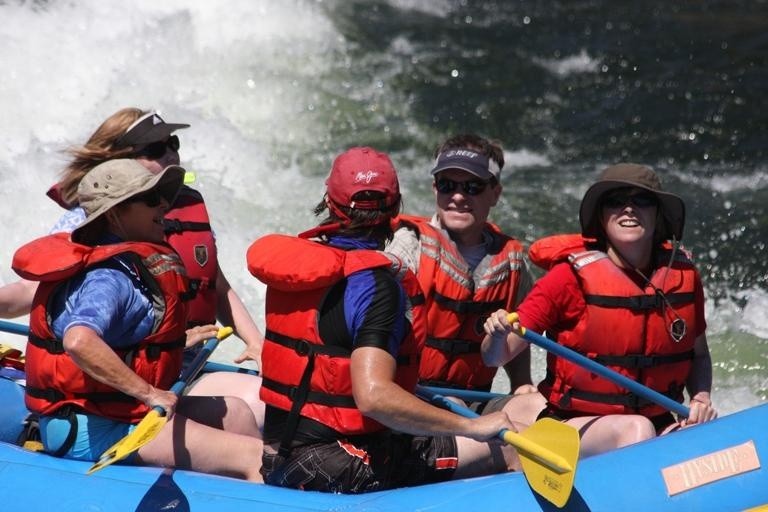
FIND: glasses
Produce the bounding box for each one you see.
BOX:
[436,176,485,195]
[123,190,160,207]
[130,135,180,159]
[605,193,656,209]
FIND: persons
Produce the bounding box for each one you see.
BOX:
[480,162,713,459]
[381,133,533,416]
[12,157,278,483]
[247,146,530,495]
[1,106,265,430]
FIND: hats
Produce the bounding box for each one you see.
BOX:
[326,145,401,210]
[115,112,191,143]
[430,149,501,180]
[578,163,685,243]
[71,158,186,243]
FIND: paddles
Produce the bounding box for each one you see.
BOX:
[412,380,581,507]
[85,324,235,475]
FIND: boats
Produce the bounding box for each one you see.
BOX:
[0,359,767,512]
